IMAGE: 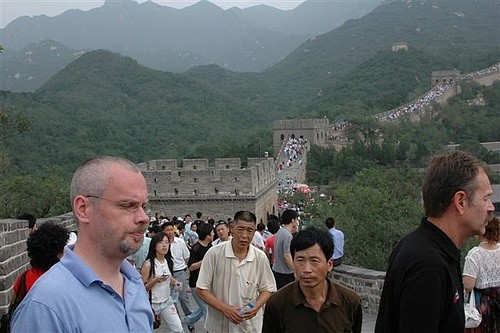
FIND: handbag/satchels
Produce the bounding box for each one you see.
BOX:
[464,288,482,328]
[0,270,30,333]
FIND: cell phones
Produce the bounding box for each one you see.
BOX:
[163,274,169,277]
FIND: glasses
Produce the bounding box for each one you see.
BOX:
[215,221,227,228]
[86,195,153,214]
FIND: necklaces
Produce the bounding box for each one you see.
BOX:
[479,242,493,246]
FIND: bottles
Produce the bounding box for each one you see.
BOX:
[172,283,180,302]
[238,302,254,315]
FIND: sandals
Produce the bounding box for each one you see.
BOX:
[188,324,195,333]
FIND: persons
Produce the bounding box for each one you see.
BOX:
[12,220,70,315]
[66,211,323,328]
[10,156,154,333]
[211,223,234,247]
[463,217,500,333]
[276,136,308,173]
[374,151,495,333]
[184,223,215,333]
[261,227,362,333]
[272,210,299,290]
[196,211,277,333]
[325,217,345,267]
[277,173,314,210]
[141,232,183,333]
[331,83,449,131]
[154,222,195,333]
